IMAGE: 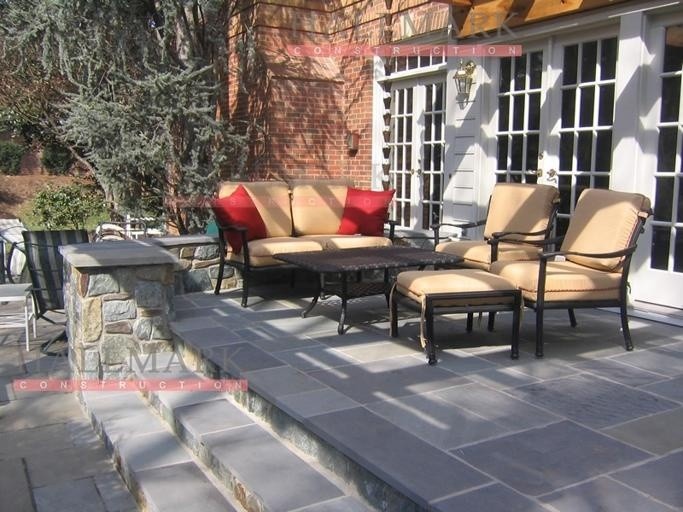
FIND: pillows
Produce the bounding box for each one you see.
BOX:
[210,183,395,254]
[561,189,651,271]
[484,183,560,248]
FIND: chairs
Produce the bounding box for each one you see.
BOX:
[0,218,148,356]
[430,183,562,327]
[487,188,654,357]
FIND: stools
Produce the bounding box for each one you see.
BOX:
[389,269,522,366]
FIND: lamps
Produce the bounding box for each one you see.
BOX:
[453,58,475,96]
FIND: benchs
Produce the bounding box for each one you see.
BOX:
[215,182,399,308]
